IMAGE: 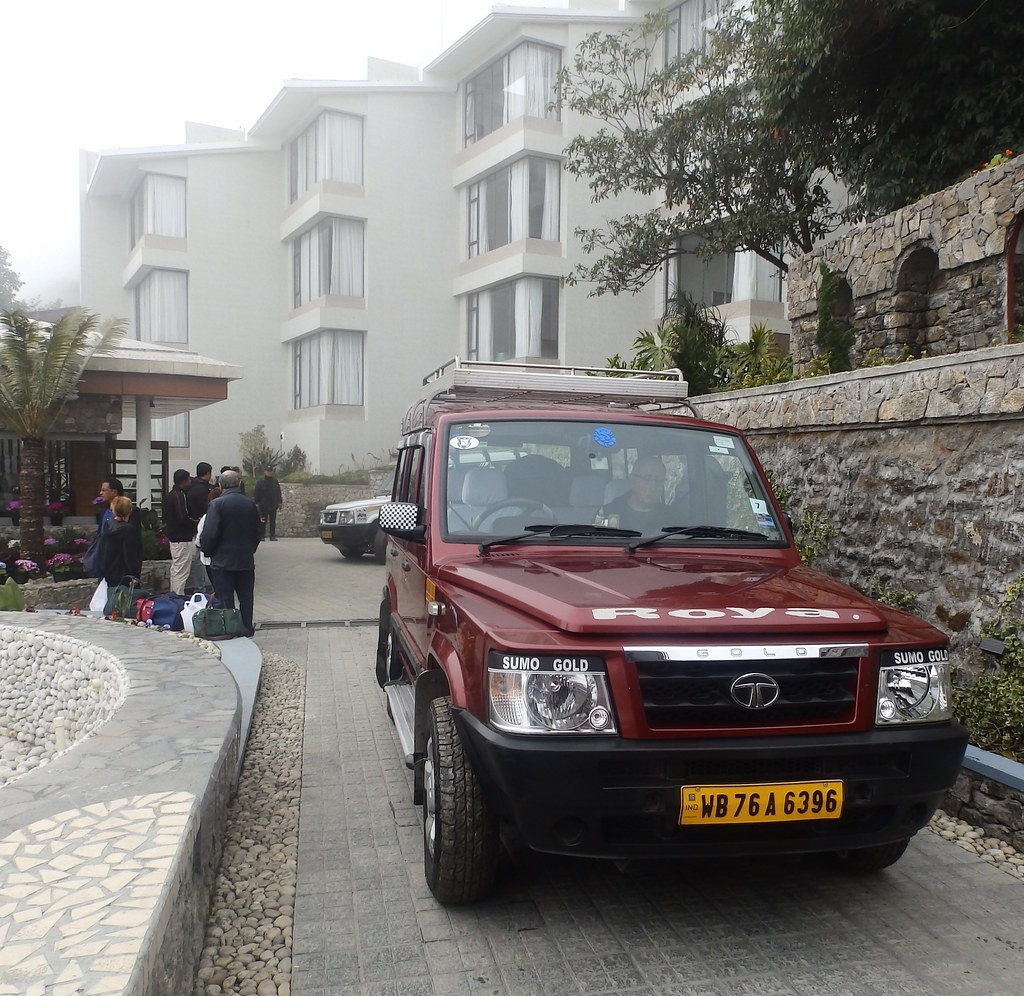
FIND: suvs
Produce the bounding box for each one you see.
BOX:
[320,451,529,559]
[374,356,971,901]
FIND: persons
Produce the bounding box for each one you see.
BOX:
[592,454,677,537]
[253,465,283,541]
[163,469,201,598]
[199,469,262,639]
[194,487,223,594]
[99,495,142,589]
[97,478,125,536]
[232,466,247,496]
[186,461,215,525]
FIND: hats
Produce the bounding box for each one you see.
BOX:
[264,465,273,470]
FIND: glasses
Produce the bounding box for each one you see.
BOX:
[633,472,670,485]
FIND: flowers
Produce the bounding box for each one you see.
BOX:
[48,500,66,515]
[43,538,60,550]
[93,496,108,505]
[15,558,40,581]
[8,501,20,509]
[0,562,7,577]
[47,552,74,572]
[6,539,21,553]
[74,552,86,569]
[73,538,91,551]
[158,537,170,550]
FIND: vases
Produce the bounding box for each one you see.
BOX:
[96,505,109,525]
[52,570,76,582]
[50,513,63,525]
[11,510,20,525]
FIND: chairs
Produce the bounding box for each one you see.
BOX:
[601,477,632,505]
[445,468,473,504]
[446,464,522,534]
[551,475,604,525]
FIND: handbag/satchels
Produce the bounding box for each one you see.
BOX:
[192,608,246,640]
[103,575,136,617]
[180,593,208,634]
[82,531,104,578]
[135,598,156,622]
[150,594,184,631]
[89,578,108,612]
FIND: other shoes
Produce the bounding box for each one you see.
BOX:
[260,538,278,541]
